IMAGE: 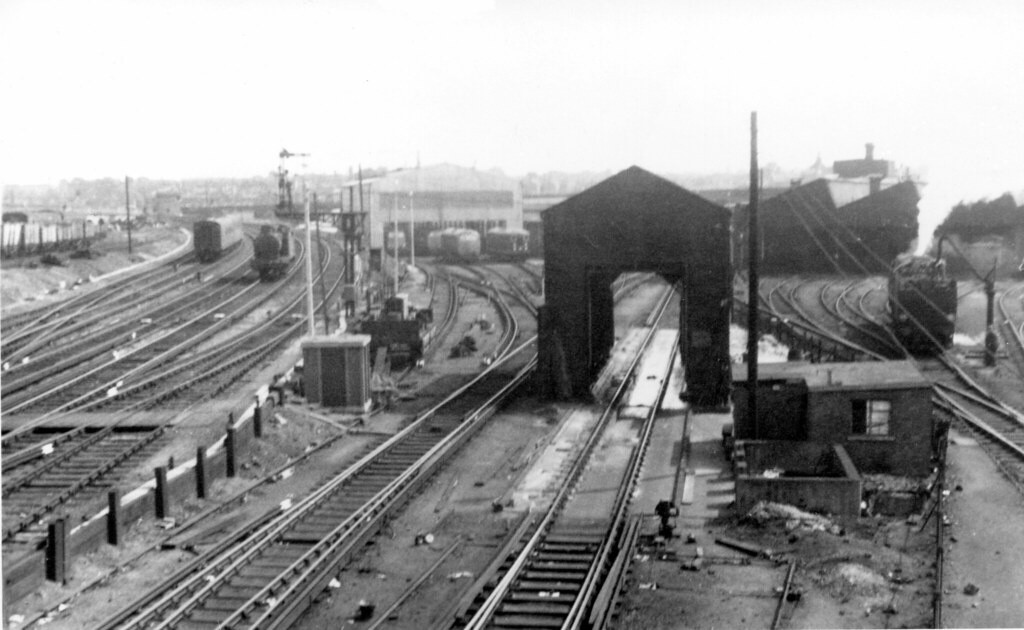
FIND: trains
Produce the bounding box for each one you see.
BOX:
[248,226,294,279]
[191,213,242,261]
[889,250,960,350]
